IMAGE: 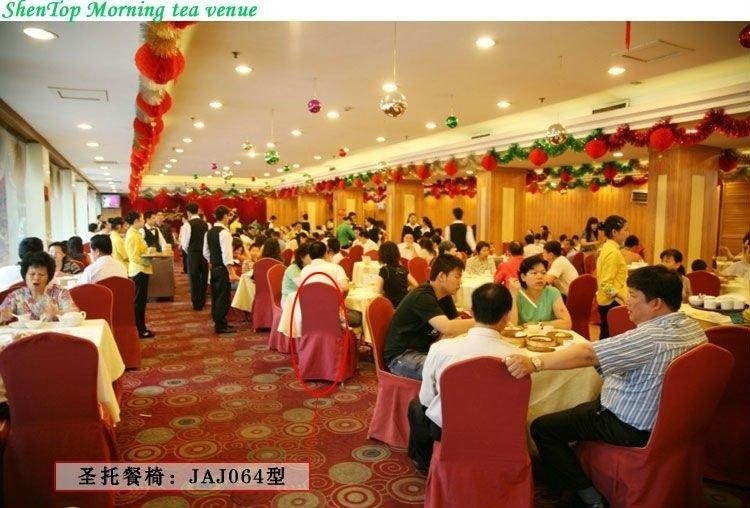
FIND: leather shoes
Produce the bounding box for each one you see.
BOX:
[217,326,237,333]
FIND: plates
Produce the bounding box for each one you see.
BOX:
[0,331,14,345]
[7,320,40,329]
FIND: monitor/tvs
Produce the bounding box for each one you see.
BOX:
[101,194,121,209]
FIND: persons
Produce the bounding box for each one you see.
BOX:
[1,203,749,508]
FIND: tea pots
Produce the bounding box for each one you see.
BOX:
[55,310,86,328]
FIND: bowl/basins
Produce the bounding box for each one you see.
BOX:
[162,244,172,256]
[24,321,41,330]
[688,295,704,306]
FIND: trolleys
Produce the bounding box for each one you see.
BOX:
[144,256,174,303]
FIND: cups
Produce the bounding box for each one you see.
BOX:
[147,247,157,256]
[527,323,554,335]
[17,314,30,326]
[702,295,745,311]
[632,261,648,269]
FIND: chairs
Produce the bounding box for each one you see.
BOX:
[2,223,179,508]
[233,251,750,508]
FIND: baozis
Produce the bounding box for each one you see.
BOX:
[503,325,523,330]
[515,331,526,337]
[555,332,566,337]
